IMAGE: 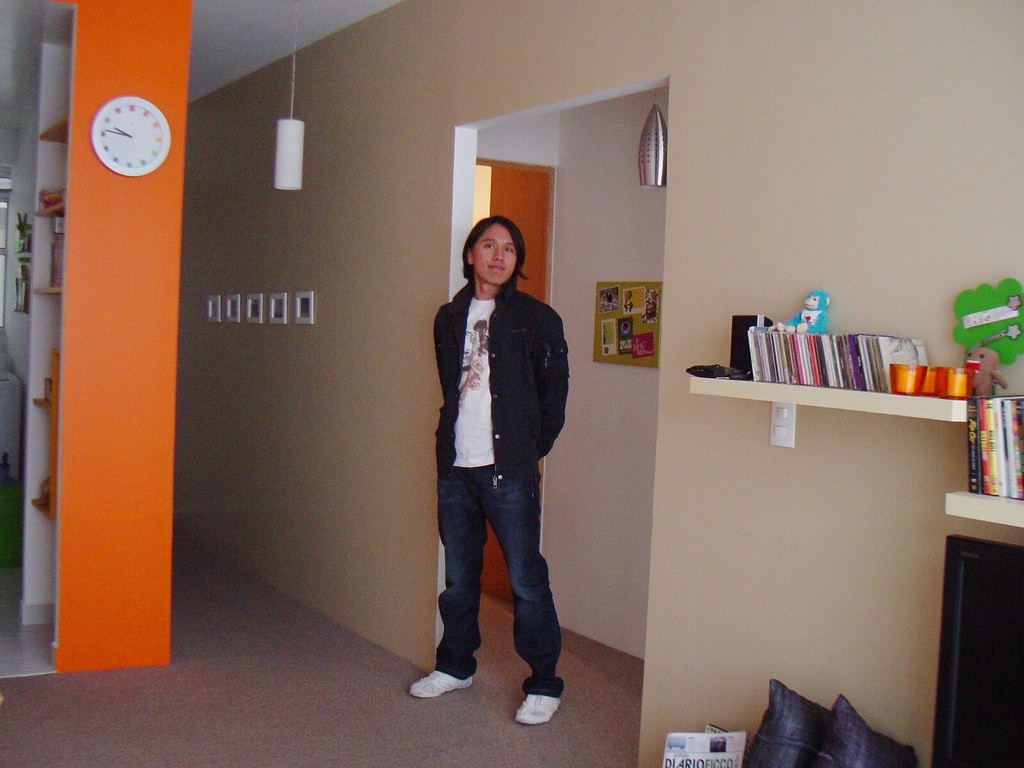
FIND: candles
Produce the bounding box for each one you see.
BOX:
[921,365,943,397]
[935,366,976,400]
[889,363,929,396]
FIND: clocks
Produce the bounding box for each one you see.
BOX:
[92,96,172,177]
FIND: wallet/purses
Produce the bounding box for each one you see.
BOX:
[686,364,749,379]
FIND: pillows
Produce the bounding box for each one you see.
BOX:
[743,678,830,768]
[811,694,916,768]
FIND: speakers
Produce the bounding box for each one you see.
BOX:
[730,315,773,379]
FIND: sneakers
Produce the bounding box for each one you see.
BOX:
[410,671,473,699]
[514,694,560,724]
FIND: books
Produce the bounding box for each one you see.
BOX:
[15,277,30,313]
[663,731,746,768]
[746,325,929,395]
[967,396,1024,500]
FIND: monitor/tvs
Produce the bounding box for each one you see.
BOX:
[929,533,1024,768]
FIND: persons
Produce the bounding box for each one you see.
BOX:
[408,215,570,724]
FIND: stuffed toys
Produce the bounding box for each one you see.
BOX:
[966,343,1009,398]
[769,288,831,334]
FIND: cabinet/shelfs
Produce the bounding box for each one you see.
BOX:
[19,0,194,672]
[687,377,1024,528]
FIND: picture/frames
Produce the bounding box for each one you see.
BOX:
[206,294,222,322]
[245,293,264,324]
[268,292,287,325]
[225,293,241,323]
[294,291,314,325]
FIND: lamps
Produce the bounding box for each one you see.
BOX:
[274,0,305,191]
[638,87,668,189]
